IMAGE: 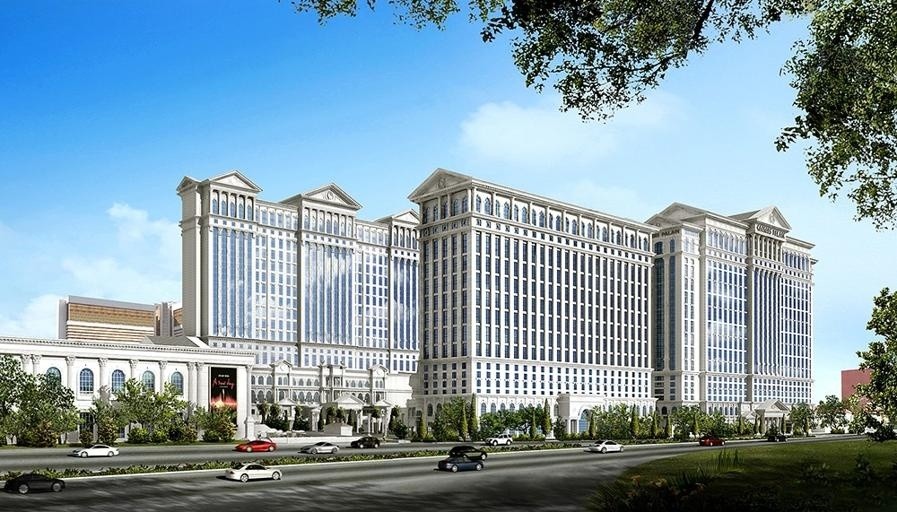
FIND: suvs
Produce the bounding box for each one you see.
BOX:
[486,434,513,446]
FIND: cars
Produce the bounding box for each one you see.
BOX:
[72,444,120,458]
[3,474,65,495]
[300,437,380,455]
[235,440,277,453]
[589,439,625,454]
[438,446,488,473]
[225,462,283,483]
[699,436,726,446]
[768,435,788,442]
